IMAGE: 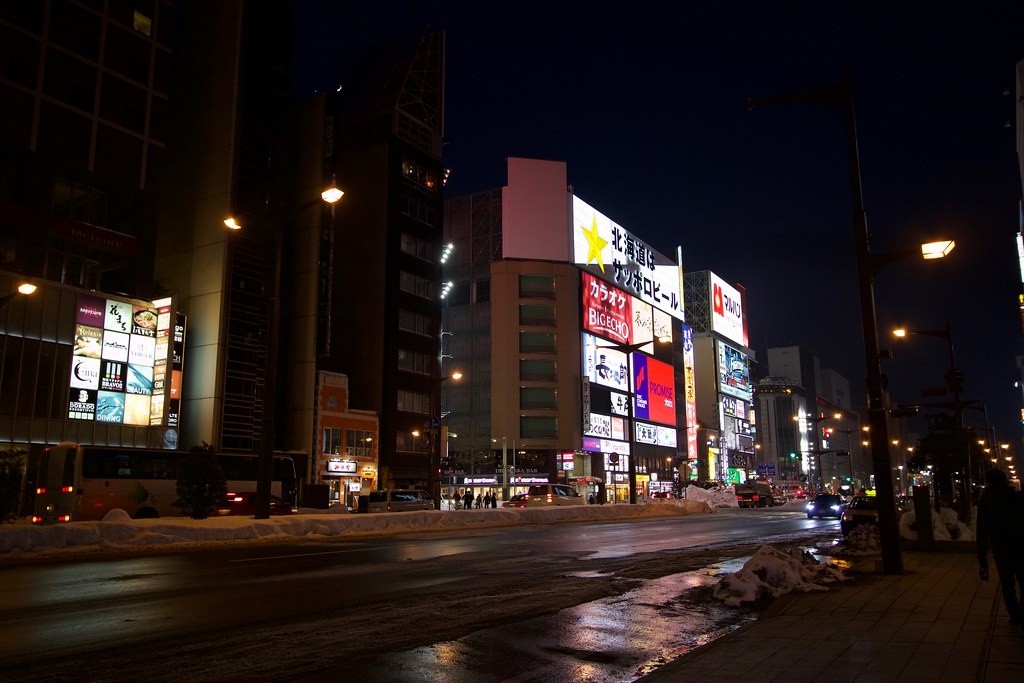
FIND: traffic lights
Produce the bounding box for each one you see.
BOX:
[790,451,796,458]
[796,454,802,461]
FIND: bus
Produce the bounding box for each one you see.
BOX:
[31,441,298,525]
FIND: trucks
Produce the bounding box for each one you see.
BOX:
[734,478,775,507]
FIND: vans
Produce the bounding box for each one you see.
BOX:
[367,490,435,513]
[525,484,585,507]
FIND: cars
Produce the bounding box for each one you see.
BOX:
[806,492,850,520]
[899,496,914,511]
[440,492,462,509]
[651,492,675,502]
[501,493,526,508]
[840,489,905,535]
[775,483,808,506]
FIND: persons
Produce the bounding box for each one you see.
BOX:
[475,494,482,510]
[462,491,469,510]
[483,492,491,509]
[466,491,474,510]
[973,469,1024,638]
[453,490,461,510]
[490,492,497,509]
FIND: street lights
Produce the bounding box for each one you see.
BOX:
[836,424,870,481]
[412,430,434,490]
[446,431,457,457]
[491,436,507,501]
[743,444,760,466]
[851,193,958,575]
[223,186,345,518]
[792,412,842,476]
[430,372,463,417]
[977,403,1018,482]
[893,419,914,464]
[594,335,669,504]
[891,321,968,524]
